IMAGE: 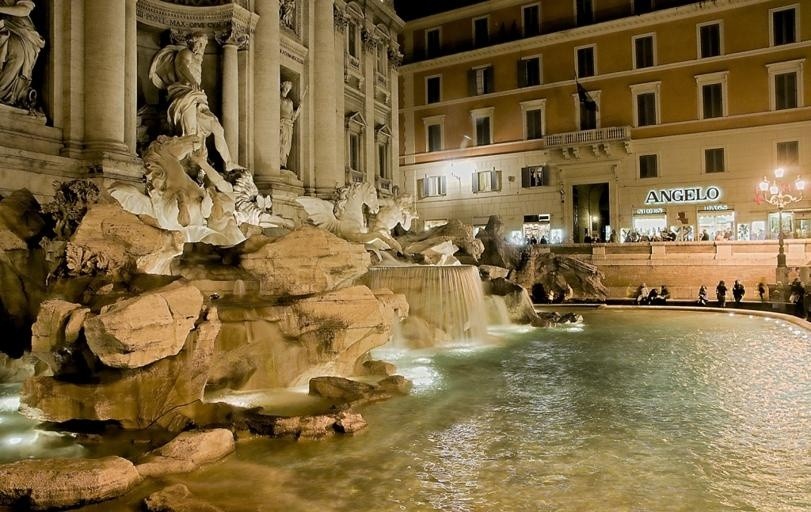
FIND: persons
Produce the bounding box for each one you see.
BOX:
[0,0,47,119]
[146,29,248,174]
[757,282,766,303]
[696,283,709,306]
[632,282,672,306]
[278,78,304,171]
[715,280,728,308]
[732,278,745,308]
[529,235,548,244]
[608,228,618,243]
[700,228,710,240]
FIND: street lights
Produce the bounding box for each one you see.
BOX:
[759,166,807,284]
